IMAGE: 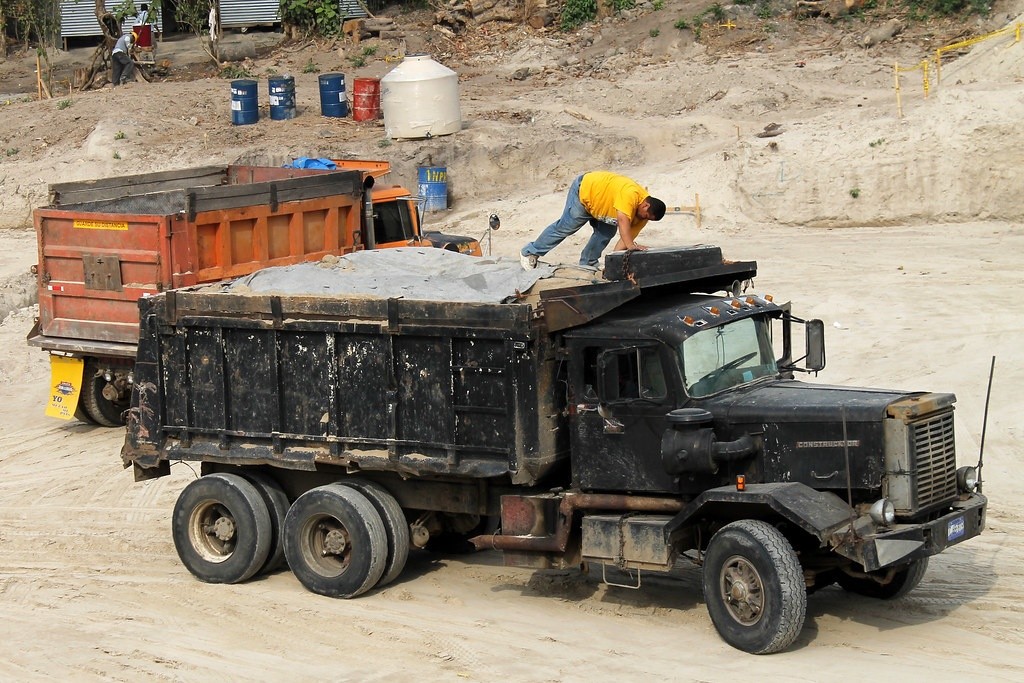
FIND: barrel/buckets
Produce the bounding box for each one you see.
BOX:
[318,72,347,118]
[268,75,296,119]
[231,80,259,124]
[417,165,448,211]
[353,77,380,121]
[133,24,151,46]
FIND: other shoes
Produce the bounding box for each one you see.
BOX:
[519,249,539,271]
[577,263,602,272]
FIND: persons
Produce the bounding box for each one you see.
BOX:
[519,169,666,272]
[132,3,161,52]
[110,31,138,86]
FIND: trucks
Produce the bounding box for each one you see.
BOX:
[27,157,501,428]
[119,244,996,655]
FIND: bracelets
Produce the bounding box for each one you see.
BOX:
[633,241,637,245]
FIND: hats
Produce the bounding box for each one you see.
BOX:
[130,32,138,43]
